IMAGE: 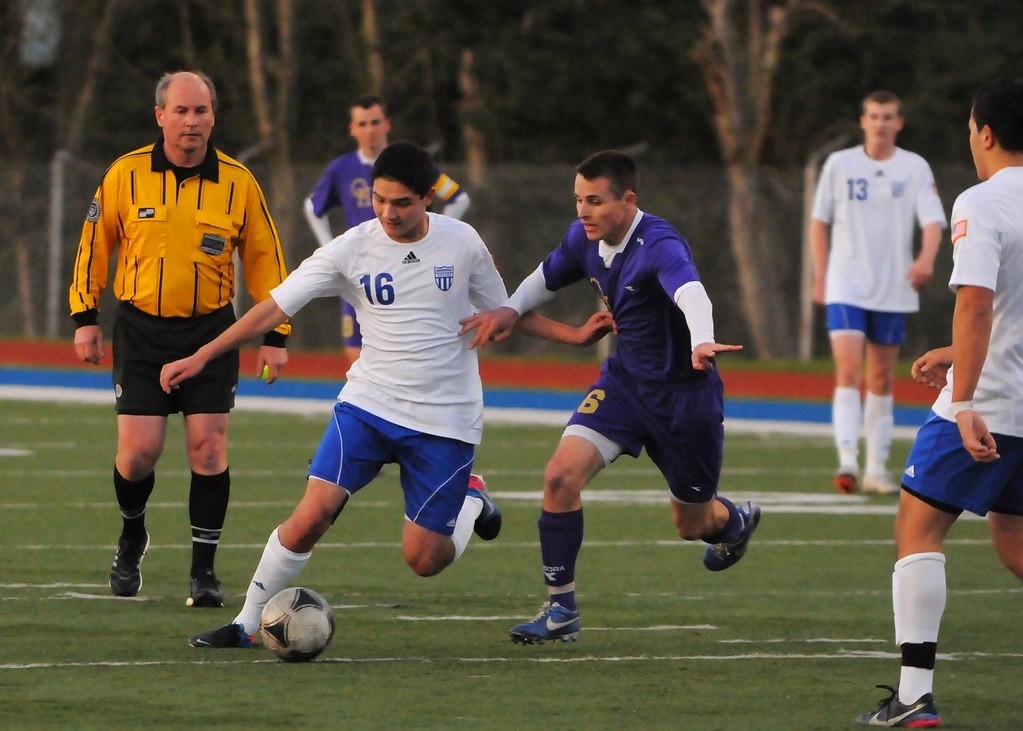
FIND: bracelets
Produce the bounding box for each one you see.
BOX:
[952,401,975,416]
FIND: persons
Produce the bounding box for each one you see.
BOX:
[160,142,502,647]
[301,97,471,363]
[807,90,949,492]
[852,71,1023,729]
[457,152,761,647]
[68,70,293,608]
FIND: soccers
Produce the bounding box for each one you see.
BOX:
[259,587,335,662]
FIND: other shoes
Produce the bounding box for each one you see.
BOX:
[836,471,856,495]
[863,475,899,494]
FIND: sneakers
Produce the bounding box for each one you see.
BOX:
[702,501,761,571]
[509,600,579,644]
[468,474,501,541]
[191,569,226,607]
[187,622,260,649]
[109,532,149,596]
[855,684,940,728]
